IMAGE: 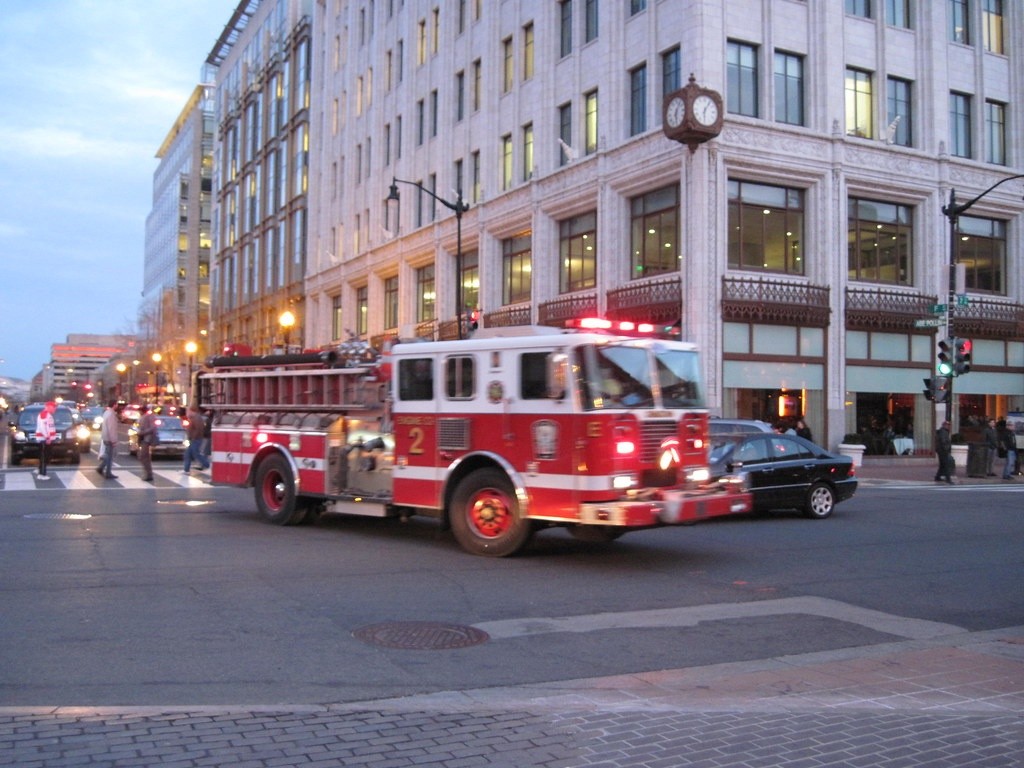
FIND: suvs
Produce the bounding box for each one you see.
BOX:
[8,403,80,466]
[708,414,777,432]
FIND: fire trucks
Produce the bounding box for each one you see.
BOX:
[189,318,752,558]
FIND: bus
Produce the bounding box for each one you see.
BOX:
[118,403,128,417]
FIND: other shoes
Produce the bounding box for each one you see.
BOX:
[178,469,190,475]
[947,479,953,485]
[33,470,39,475]
[1004,475,1014,479]
[934,478,943,480]
[37,474,50,481]
[142,476,153,481]
[96,468,103,476]
[106,474,118,478]
[987,472,996,476]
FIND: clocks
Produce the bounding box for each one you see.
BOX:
[665,97,687,128]
[692,93,719,126]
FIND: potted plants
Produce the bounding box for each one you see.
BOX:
[838,433,866,467]
[949,432,970,466]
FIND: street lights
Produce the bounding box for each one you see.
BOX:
[279,311,295,355]
[186,342,197,385]
[152,353,162,404]
[384,175,463,338]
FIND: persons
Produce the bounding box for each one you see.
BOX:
[136,407,156,479]
[778,418,812,443]
[859,422,913,449]
[34,400,57,480]
[96,399,119,480]
[181,407,211,473]
[984,416,1017,480]
[935,421,955,485]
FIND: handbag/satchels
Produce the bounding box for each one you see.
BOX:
[150,434,159,447]
[98,440,107,462]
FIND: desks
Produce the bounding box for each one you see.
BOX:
[890,438,914,455]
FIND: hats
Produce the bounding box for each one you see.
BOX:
[943,421,951,426]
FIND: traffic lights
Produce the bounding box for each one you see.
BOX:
[923,378,935,400]
[956,337,973,374]
[938,339,953,376]
[779,397,795,417]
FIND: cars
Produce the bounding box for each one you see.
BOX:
[61,400,76,409]
[710,432,858,518]
[81,407,106,431]
[121,403,190,460]
